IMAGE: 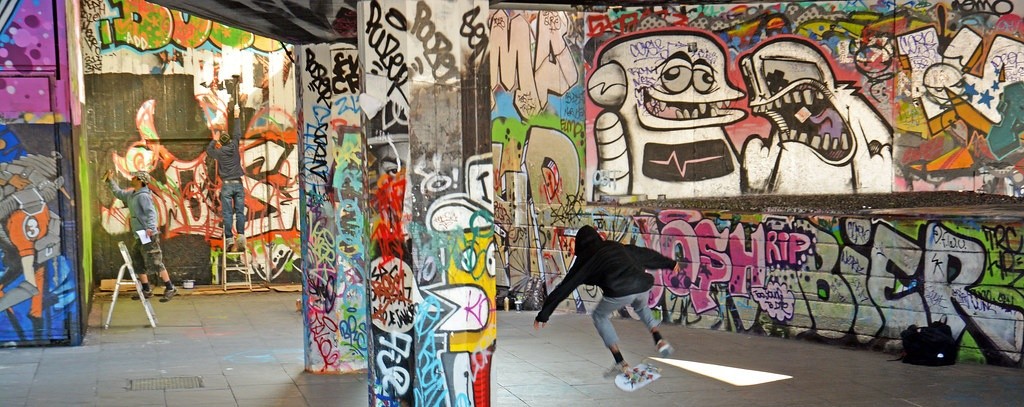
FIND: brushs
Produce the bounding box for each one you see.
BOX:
[232,74,240,105]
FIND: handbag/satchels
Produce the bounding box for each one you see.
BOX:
[887,314,960,367]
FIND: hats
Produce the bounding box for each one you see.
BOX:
[220,132,230,145]
[129,171,150,184]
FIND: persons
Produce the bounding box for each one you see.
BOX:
[106,169,178,303]
[206,105,247,252]
[534,225,678,378]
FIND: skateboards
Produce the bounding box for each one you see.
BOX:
[614,365,663,391]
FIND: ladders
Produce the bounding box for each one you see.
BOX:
[222,224,252,292]
[104,240,158,330]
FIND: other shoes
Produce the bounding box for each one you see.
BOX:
[159,285,178,302]
[603,360,629,378]
[657,340,674,356]
[226,238,234,252]
[236,234,245,252]
[131,288,154,300]
